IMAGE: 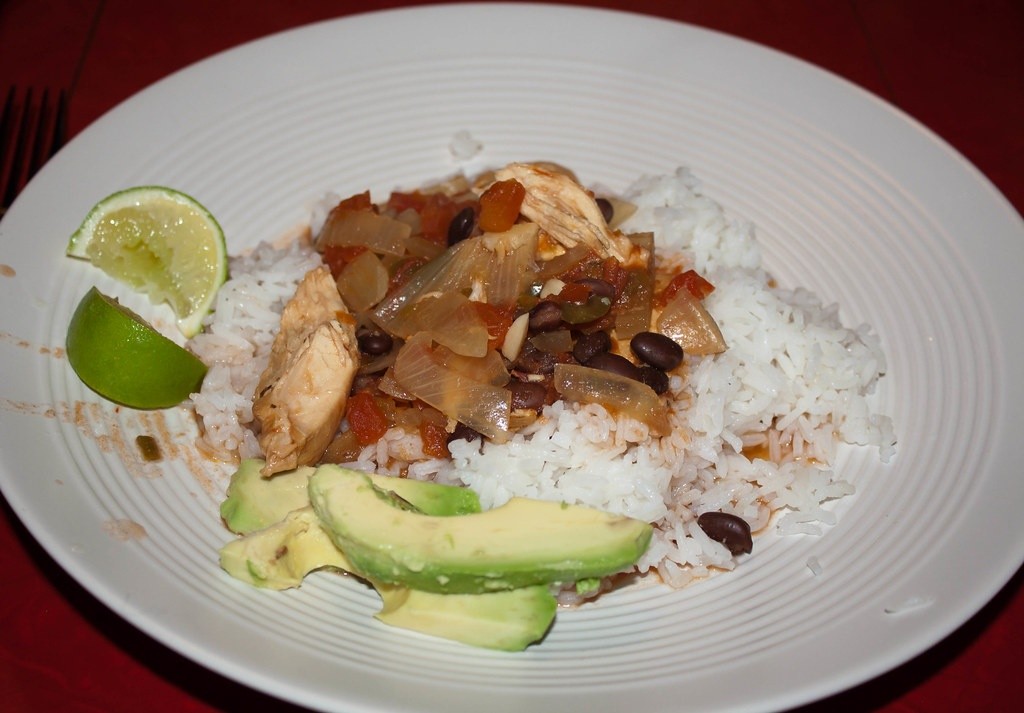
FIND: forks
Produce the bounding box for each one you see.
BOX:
[0,83,71,213]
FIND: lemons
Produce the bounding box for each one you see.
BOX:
[63,184,227,409]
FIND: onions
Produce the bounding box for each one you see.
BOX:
[340,207,725,448]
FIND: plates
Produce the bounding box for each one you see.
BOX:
[0,3,1024,713]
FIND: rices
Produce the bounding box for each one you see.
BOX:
[198,218,899,580]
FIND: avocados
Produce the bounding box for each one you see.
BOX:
[221,453,651,652]
